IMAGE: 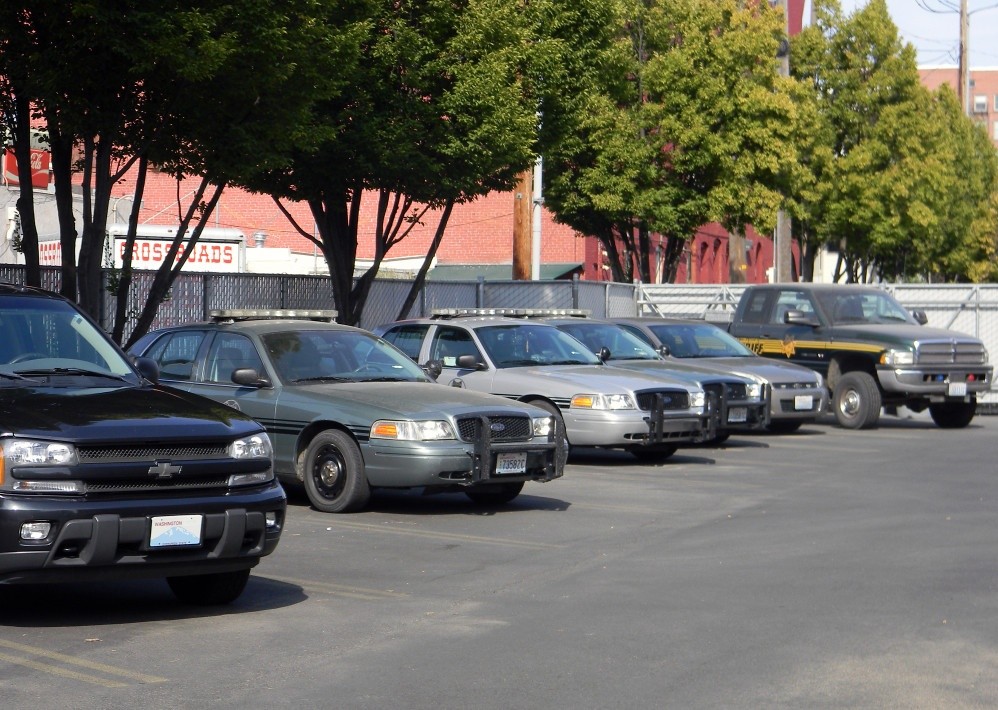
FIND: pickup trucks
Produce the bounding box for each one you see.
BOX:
[670,283,993,429]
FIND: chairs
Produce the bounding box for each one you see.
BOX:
[219,347,244,378]
[776,304,796,323]
[493,340,525,362]
[586,336,604,353]
[833,300,865,319]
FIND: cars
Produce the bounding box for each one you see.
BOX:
[507,309,771,446]
[597,318,828,435]
[117,309,568,514]
[0,283,287,604]
[350,309,718,461]
